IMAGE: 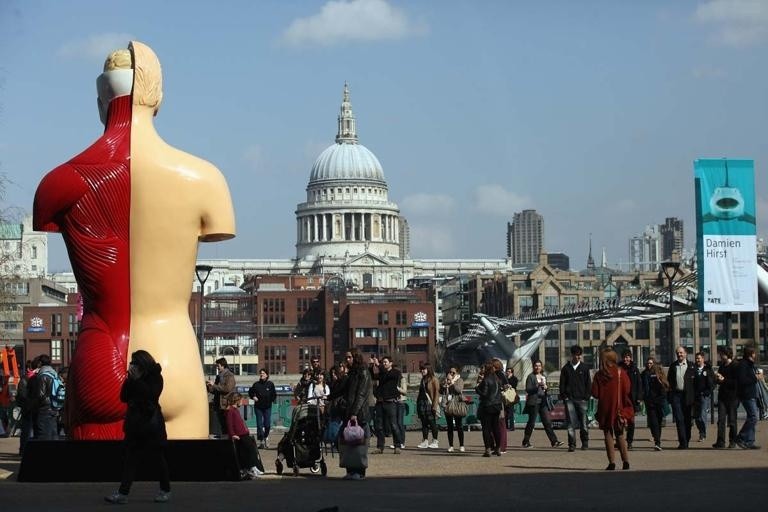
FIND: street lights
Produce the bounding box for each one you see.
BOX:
[661,261,681,365]
[192,262,215,367]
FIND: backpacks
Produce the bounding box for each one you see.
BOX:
[49,376,67,410]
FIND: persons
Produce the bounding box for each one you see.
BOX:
[249,369,277,449]
[417,362,440,448]
[522,360,565,449]
[33,39,236,440]
[592,348,632,470]
[475,359,519,457]
[613,345,716,451]
[104,350,172,504]
[439,367,465,452]
[295,347,407,481]
[559,345,592,451]
[712,347,741,448]
[206,358,236,438]
[734,346,764,449]
[0,354,69,457]
[225,392,264,479]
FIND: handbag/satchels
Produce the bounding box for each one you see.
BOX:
[614,412,627,436]
[323,420,341,442]
[502,386,520,407]
[435,404,441,419]
[343,418,365,445]
[445,400,468,417]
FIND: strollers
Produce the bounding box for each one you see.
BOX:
[274,398,328,476]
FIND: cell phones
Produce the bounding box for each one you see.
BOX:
[370,353,375,358]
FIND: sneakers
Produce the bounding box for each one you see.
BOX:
[352,473,366,481]
[343,473,352,481]
[417,439,429,449]
[734,434,748,449]
[400,444,406,449]
[729,439,736,449]
[250,465,265,475]
[247,468,258,477]
[712,442,724,449]
[264,438,269,448]
[747,439,762,449]
[623,462,629,470]
[522,441,533,448]
[427,439,440,449]
[448,447,454,453]
[104,491,128,505]
[389,445,395,449]
[606,464,615,470]
[459,446,466,452]
[371,448,383,454]
[394,447,401,454]
[568,444,576,451]
[155,490,173,503]
[552,441,564,448]
[581,445,589,449]
[259,440,264,449]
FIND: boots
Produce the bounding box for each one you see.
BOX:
[492,447,500,456]
[627,440,632,450]
[483,447,491,457]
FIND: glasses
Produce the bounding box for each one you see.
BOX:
[449,371,456,374]
[312,361,319,364]
